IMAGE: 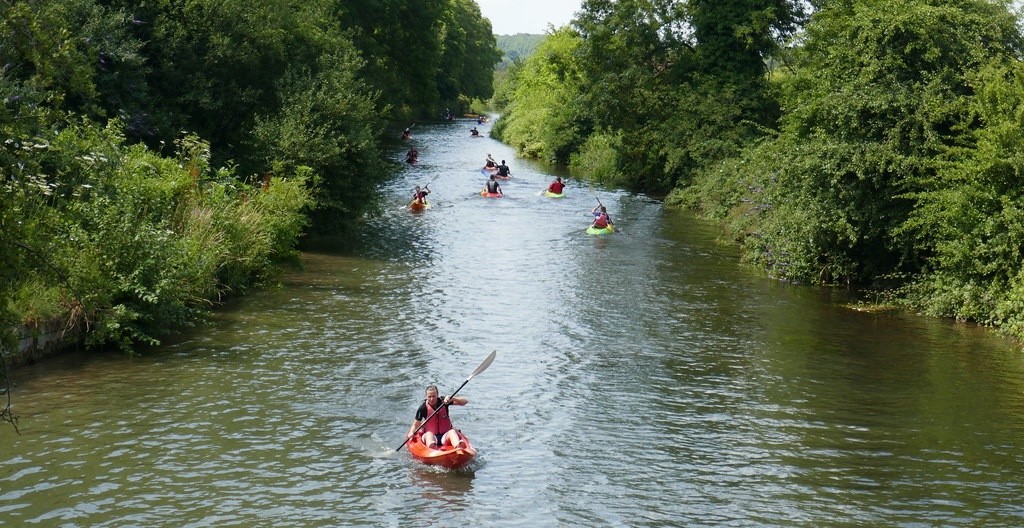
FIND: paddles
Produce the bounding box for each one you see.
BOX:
[540,174,576,195]
[588,183,620,234]
[395,348,499,452]
[404,173,440,208]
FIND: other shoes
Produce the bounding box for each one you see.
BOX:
[455,440,467,449]
[428,441,437,450]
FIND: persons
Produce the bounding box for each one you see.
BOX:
[407,147,418,158]
[403,128,410,136]
[485,154,494,167]
[408,386,468,448]
[549,177,565,194]
[486,175,499,193]
[471,127,478,135]
[413,186,430,198]
[592,204,610,229]
[496,160,510,177]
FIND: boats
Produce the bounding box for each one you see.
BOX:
[544,190,566,199]
[401,136,413,144]
[470,134,479,138]
[493,172,511,181]
[410,196,430,211]
[485,166,498,172]
[404,158,420,166]
[405,421,477,469]
[586,223,613,235]
[480,187,504,200]
